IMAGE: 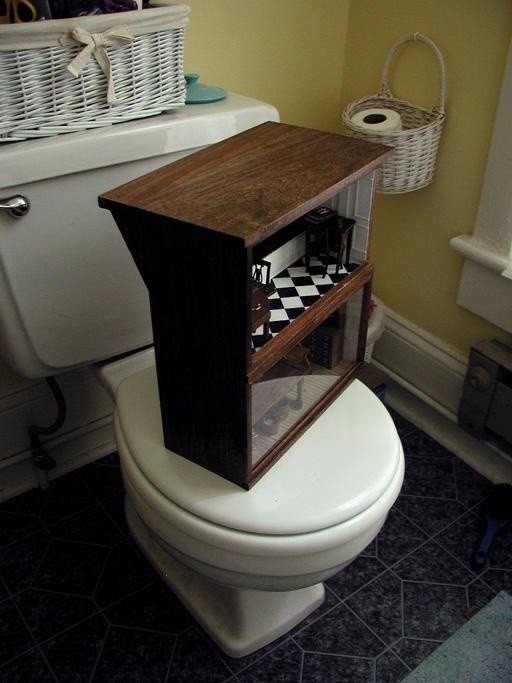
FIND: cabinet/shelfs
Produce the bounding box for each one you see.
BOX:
[96,121,397,491]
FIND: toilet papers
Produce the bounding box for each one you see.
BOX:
[351,109,401,132]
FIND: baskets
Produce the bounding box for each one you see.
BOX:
[0,2,191,142]
[340,32,446,196]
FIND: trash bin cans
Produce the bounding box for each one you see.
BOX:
[360,302,391,373]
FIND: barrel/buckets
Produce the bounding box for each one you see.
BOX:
[1,87,405,657]
